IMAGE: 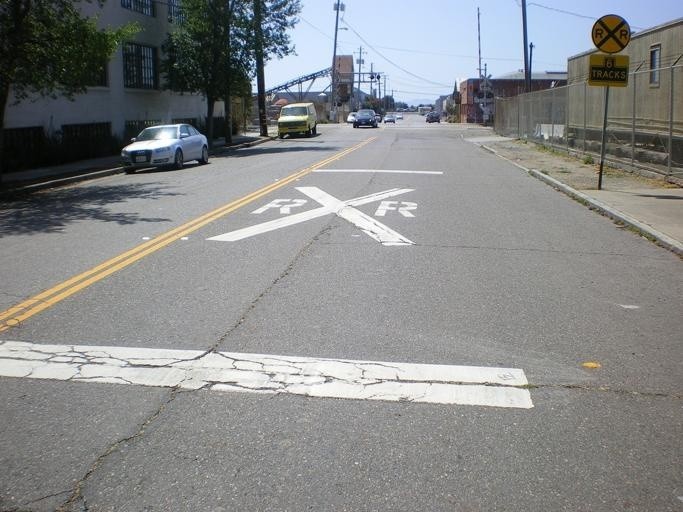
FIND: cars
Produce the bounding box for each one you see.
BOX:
[121,123,210,172]
[346,107,403,123]
[351,109,378,128]
[396,108,407,112]
[404,108,415,112]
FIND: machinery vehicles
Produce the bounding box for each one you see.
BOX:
[267,97,288,118]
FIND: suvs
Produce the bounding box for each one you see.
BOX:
[423,112,441,124]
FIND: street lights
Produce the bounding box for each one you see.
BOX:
[336,27,348,31]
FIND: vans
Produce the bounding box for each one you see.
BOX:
[277,102,318,139]
